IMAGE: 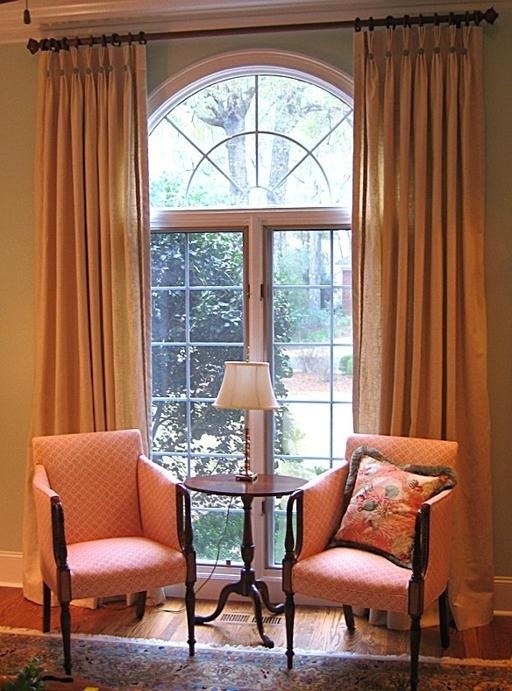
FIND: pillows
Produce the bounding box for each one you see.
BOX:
[324,445,458,570]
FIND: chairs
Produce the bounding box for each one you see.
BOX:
[32,428,196,675]
[282,433,459,690]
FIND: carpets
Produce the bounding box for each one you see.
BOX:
[0,626,512,691]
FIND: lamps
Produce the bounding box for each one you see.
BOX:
[211,346,280,482]
